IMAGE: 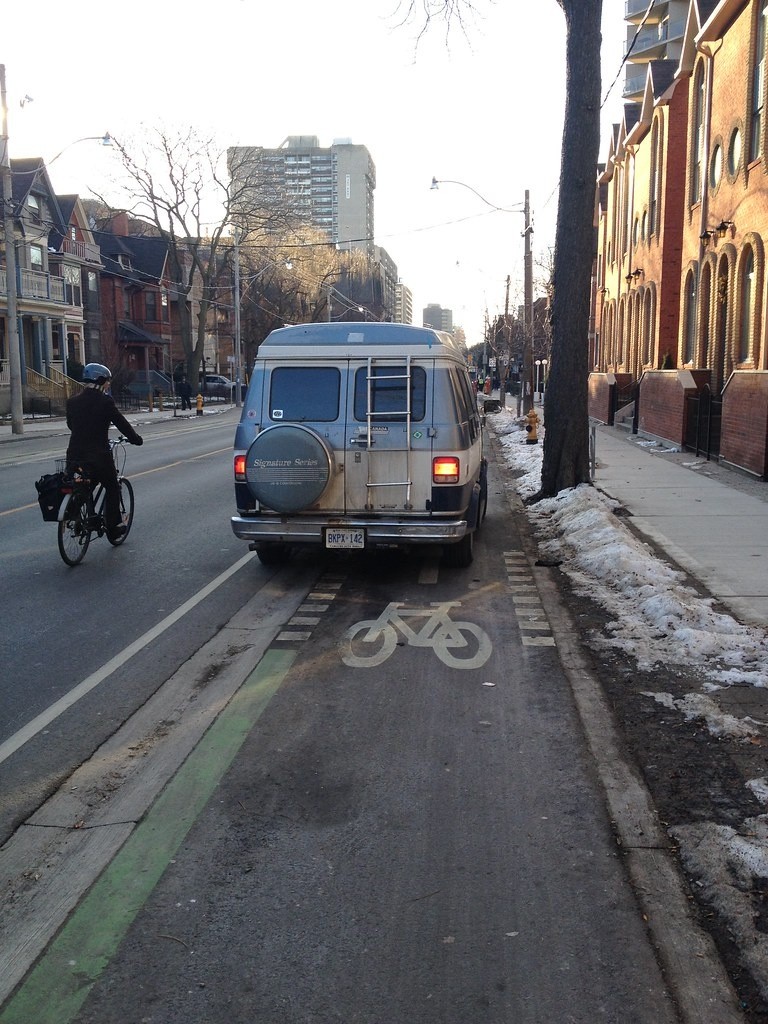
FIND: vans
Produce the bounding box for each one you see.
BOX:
[226,320,489,570]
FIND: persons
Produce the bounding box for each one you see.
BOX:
[478,378,483,391]
[179,379,192,411]
[65,363,144,542]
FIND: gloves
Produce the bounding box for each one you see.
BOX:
[135,435,143,445]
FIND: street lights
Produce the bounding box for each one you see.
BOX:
[2,133,113,434]
[428,175,536,420]
[234,259,293,407]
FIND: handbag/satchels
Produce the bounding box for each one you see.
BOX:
[35,472,64,521]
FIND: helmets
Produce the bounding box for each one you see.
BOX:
[82,363,111,385]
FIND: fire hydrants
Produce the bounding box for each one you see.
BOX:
[483,374,491,394]
[524,410,541,445]
[195,393,204,415]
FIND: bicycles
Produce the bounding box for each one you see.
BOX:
[51,435,136,567]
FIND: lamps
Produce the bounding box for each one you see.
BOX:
[698,219,734,247]
[601,286,608,294]
[626,267,643,284]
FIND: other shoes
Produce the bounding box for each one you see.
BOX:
[109,524,128,540]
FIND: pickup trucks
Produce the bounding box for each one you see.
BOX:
[199,374,247,401]
